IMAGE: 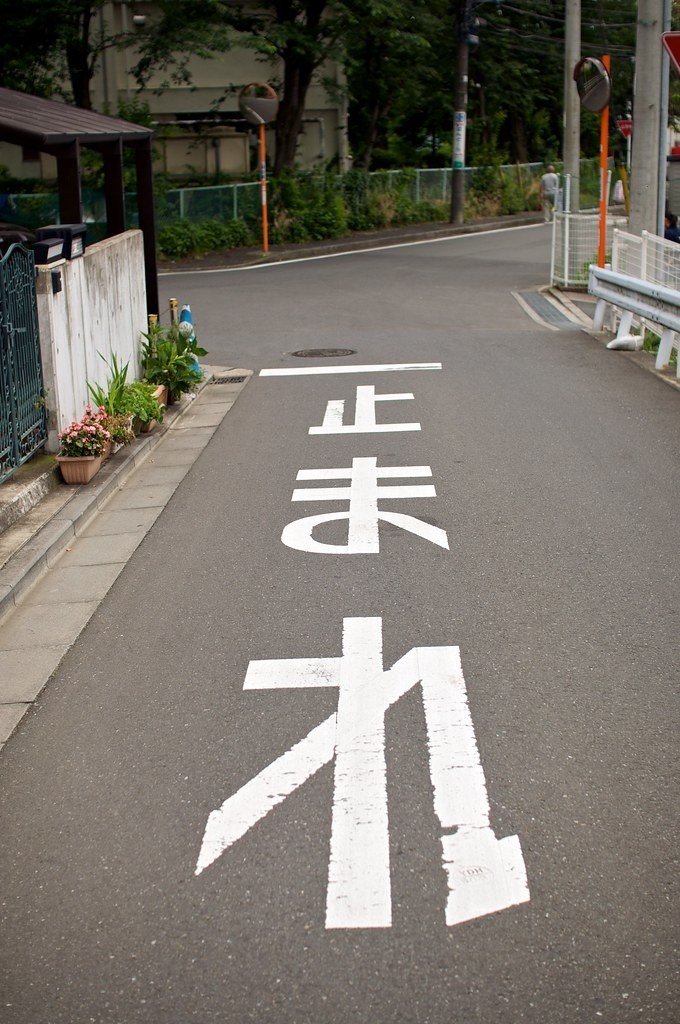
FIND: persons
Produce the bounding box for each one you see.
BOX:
[540,165,558,230]
[664,199,680,263]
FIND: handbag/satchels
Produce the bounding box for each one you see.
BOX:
[538,199,543,212]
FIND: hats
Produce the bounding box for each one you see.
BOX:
[545,165,554,172]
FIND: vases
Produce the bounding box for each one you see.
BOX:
[56,438,113,484]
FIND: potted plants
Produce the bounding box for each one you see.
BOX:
[85,321,207,453]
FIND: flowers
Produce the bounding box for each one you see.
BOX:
[57,405,115,457]
